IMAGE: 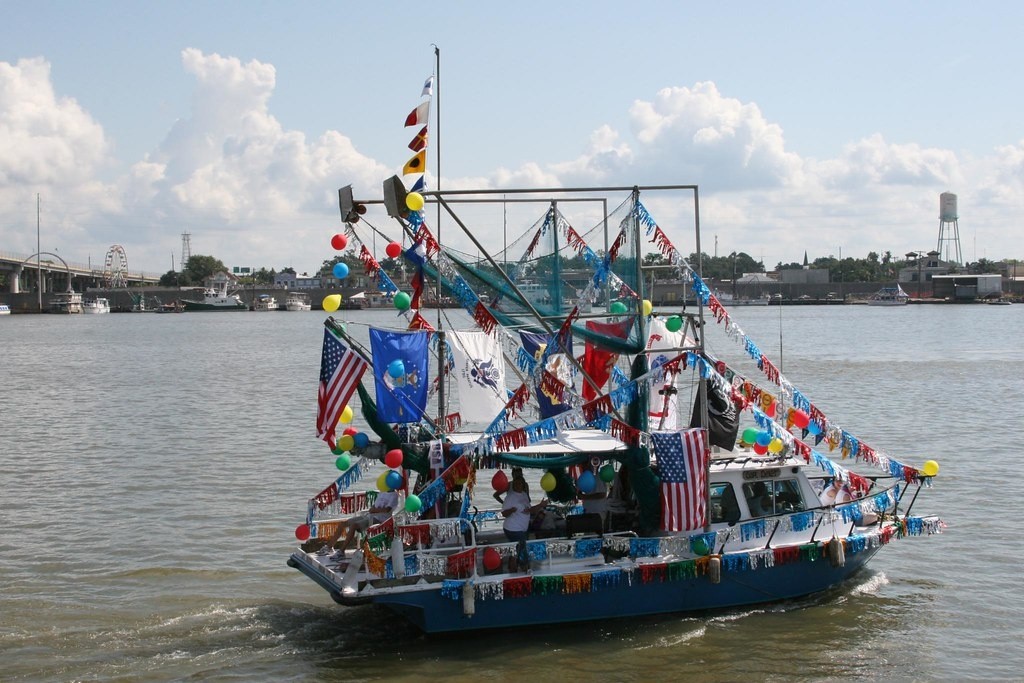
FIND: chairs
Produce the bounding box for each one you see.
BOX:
[566,511,609,562]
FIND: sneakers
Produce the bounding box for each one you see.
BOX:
[330,550,345,561]
[315,545,335,555]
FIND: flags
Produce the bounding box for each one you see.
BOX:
[315,327,749,452]
[651,427,709,532]
[403,76,434,178]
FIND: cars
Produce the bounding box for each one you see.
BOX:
[798,294,810,299]
[825,292,837,299]
[771,294,783,299]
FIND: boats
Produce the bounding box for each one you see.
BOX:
[867,281,910,306]
[0,304,11,315]
[515,283,550,305]
[153,305,181,313]
[286,174,944,636]
[254,294,280,311]
[986,300,1012,305]
[180,289,250,312]
[285,299,312,311]
[82,297,110,314]
[49,292,82,313]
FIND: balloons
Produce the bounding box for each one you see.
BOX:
[322,294,341,312]
[540,471,557,492]
[294,524,310,540]
[394,291,411,309]
[742,427,783,455]
[385,242,402,258]
[600,464,615,481]
[376,448,421,512]
[792,408,826,435]
[406,192,424,210]
[332,262,349,279]
[491,469,508,491]
[330,233,348,251]
[332,405,369,471]
[923,459,939,476]
[483,547,501,570]
[576,469,597,493]
[610,299,683,332]
[692,537,709,556]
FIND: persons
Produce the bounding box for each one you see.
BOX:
[493,468,550,574]
[316,491,398,562]
[818,475,880,527]
[722,481,772,522]
[577,467,607,532]
[606,463,639,536]
